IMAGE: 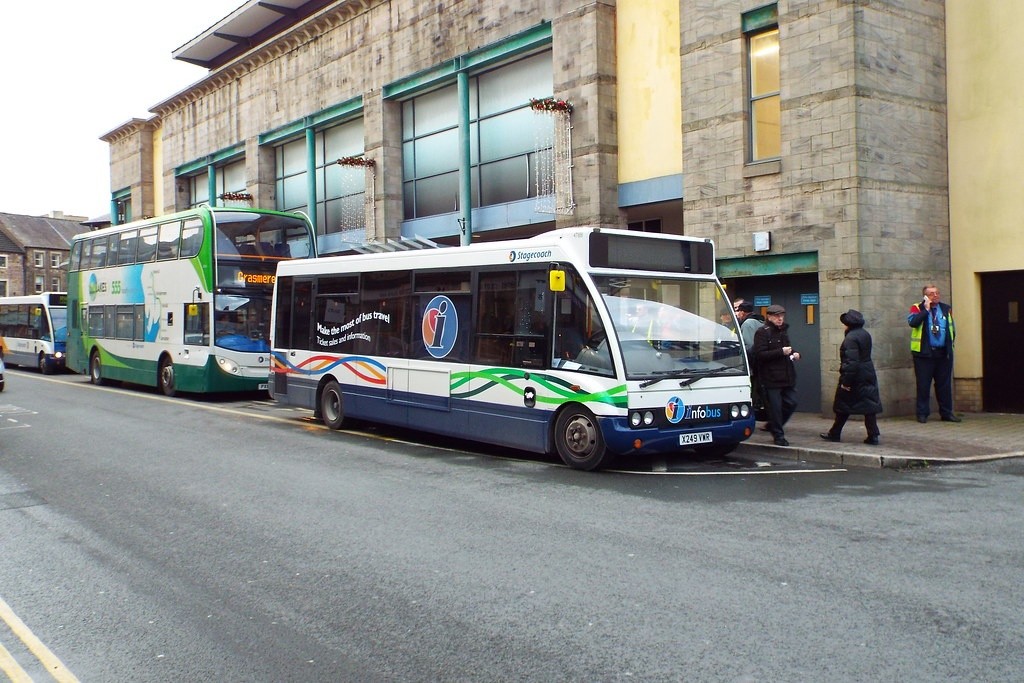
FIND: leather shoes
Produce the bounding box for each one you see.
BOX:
[941,416,961,422]
[764,423,771,431]
[820,433,831,440]
[774,439,789,446]
[918,418,926,423]
[864,435,878,445]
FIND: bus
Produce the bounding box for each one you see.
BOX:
[64,205,316,398]
[266,223,758,474]
[0,290,68,393]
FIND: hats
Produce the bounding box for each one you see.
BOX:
[720,308,731,317]
[737,303,754,313]
[840,309,865,328]
[766,305,786,315]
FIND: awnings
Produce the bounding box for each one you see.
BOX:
[171,0,338,69]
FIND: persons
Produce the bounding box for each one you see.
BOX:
[719,298,766,423]
[819,309,883,445]
[754,305,801,446]
[907,285,962,424]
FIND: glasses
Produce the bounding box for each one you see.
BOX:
[926,291,939,296]
